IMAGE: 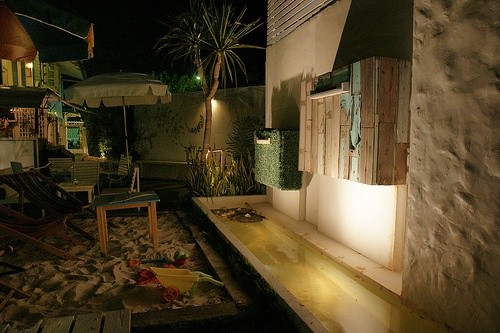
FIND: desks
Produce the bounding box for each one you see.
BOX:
[92,190,159,256]
[57,181,96,204]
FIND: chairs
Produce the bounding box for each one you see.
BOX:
[0,154,133,260]
